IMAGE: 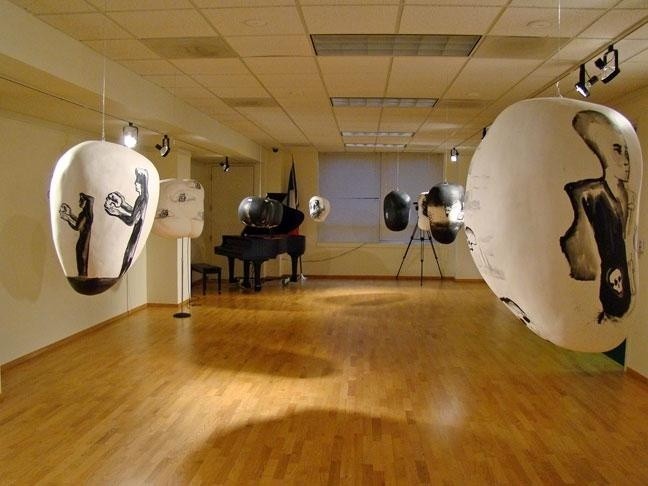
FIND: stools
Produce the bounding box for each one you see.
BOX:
[190,261,222,296]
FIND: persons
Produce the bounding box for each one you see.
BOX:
[58,192,96,276]
[105,165,150,279]
[557,109,638,283]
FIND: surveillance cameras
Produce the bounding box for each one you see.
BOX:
[272,147,278,153]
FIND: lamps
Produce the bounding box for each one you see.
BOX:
[450,145,461,161]
[154,134,171,158]
[573,42,621,99]
[219,155,230,174]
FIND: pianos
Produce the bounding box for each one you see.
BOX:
[214,193,306,292]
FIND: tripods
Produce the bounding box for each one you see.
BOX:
[396,223,443,286]
